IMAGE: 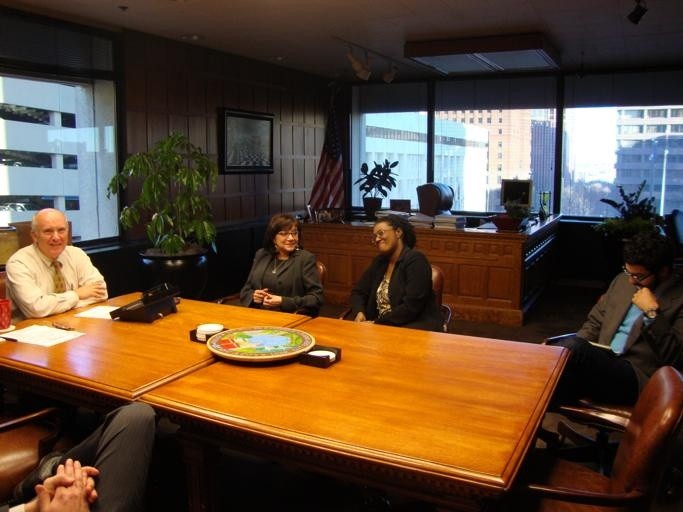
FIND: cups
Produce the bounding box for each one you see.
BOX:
[0,299,12,331]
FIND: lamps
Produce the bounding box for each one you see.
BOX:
[404,33,562,78]
[330,35,405,84]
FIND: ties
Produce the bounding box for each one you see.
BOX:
[51,261,65,293]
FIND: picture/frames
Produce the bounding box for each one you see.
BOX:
[217,107,276,174]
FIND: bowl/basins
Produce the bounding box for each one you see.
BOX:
[316,207,345,223]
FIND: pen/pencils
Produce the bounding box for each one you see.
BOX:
[0,336,16,342]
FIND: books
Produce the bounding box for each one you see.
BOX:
[375,211,465,231]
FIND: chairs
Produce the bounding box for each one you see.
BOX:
[215,261,327,317]
[337,264,453,332]
[0,272,6,299]
[417,183,454,216]
[540,332,634,418]
[0,406,74,506]
[527,365,683,512]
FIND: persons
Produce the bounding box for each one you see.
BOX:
[349,214,442,332]
[5,207,108,321]
[1,401,155,512]
[548,232,683,409]
[232,212,326,313]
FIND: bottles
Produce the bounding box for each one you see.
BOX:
[539,202,548,220]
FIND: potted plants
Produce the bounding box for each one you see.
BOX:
[106,131,219,301]
[354,159,399,210]
[488,192,537,232]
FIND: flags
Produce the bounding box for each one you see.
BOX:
[308,105,348,213]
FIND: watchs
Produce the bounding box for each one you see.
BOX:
[643,307,660,320]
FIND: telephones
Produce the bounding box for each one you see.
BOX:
[109,282,181,324]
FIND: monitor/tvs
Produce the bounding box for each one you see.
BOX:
[500,179,532,209]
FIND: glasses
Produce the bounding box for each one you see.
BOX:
[277,231,298,237]
[371,230,383,243]
[622,265,654,281]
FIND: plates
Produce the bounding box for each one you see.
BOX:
[195,324,225,341]
[309,349,336,361]
[206,325,316,365]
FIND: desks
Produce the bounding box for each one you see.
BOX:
[0,291,313,428]
[136,316,573,512]
[300,214,563,326]
